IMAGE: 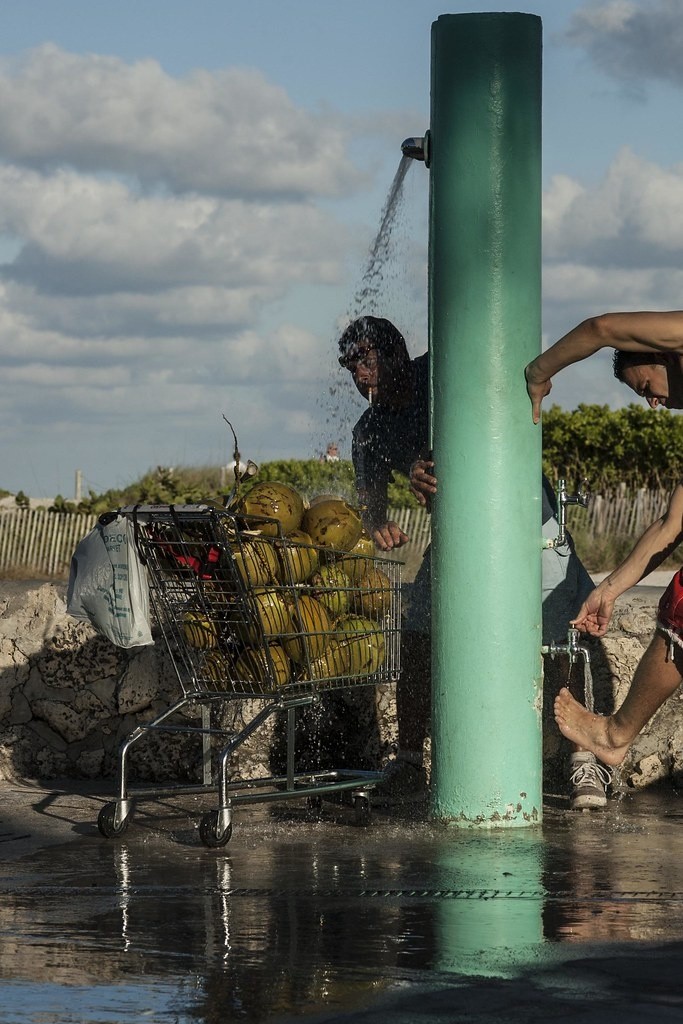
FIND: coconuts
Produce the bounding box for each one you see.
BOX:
[184,485,389,687]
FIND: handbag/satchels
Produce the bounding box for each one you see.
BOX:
[64,509,157,649]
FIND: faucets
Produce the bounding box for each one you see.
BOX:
[548,619,592,667]
[557,476,594,518]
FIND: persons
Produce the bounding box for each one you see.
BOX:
[336,313,616,811]
[319,442,341,464]
[524,306,683,768]
[224,451,248,491]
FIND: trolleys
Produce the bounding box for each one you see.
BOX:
[87,503,406,848]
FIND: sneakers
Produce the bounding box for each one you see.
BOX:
[563,750,608,808]
[370,755,427,805]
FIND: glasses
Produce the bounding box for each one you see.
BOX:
[338,343,386,368]
